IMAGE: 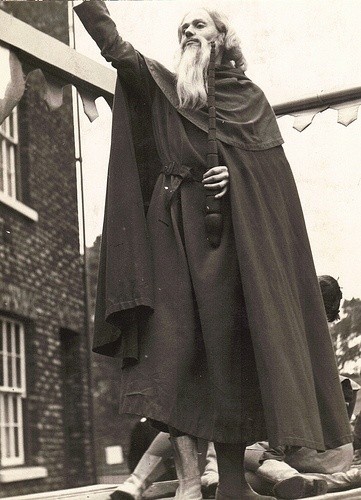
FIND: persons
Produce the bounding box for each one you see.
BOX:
[67,0,355,500]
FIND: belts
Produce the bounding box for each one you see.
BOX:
[160,164,203,210]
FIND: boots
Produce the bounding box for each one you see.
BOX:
[255,458,328,499]
[169,435,203,500]
[214,442,275,500]
[109,430,175,500]
